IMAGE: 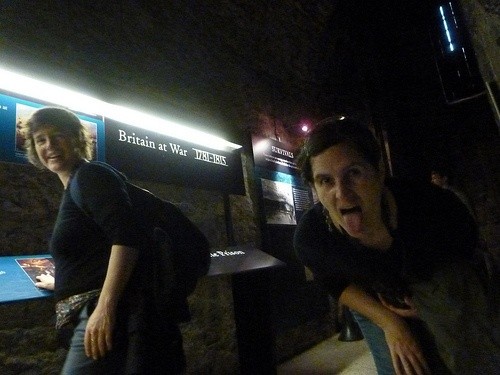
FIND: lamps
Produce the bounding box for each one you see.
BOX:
[0,67,243,153]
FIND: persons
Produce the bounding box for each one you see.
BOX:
[429,164,472,215]
[24,105,191,375]
[295,116,499,375]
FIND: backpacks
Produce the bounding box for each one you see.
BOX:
[127,182,209,322]
